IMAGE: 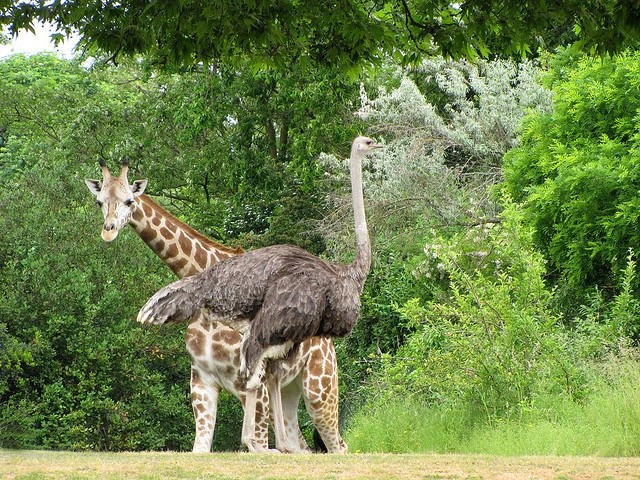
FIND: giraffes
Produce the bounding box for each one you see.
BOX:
[84,155,350,454]
